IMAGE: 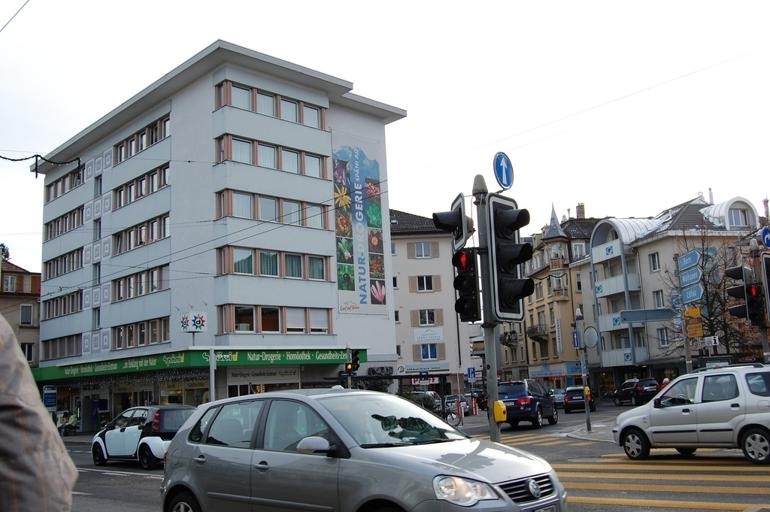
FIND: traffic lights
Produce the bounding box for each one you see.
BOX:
[346,349,360,378]
[747,282,766,328]
[723,264,753,321]
[451,248,482,322]
[488,191,537,322]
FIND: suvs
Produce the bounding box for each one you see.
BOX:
[405,389,442,412]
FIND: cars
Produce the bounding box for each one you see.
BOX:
[91,398,197,470]
[443,394,470,414]
[159,391,572,512]
[498,363,770,461]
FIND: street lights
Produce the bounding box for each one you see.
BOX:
[575,307,593,432]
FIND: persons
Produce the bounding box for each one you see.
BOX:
[0,313,80,511]
[658,377,676,400]
[60,410,77,437]
[57,411,69,433]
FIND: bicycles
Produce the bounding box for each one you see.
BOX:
[442,400,460,426]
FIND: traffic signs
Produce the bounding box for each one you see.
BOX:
[678,249,701,271]
[432,192,474,251]
[678,266,703,288]
[680,284,705,304]
